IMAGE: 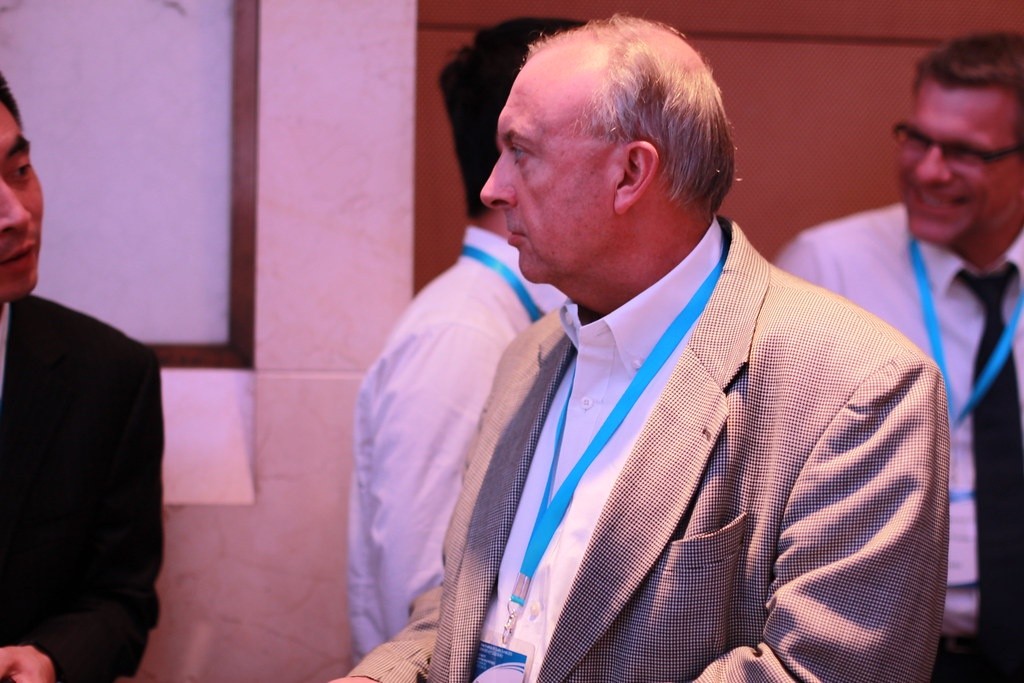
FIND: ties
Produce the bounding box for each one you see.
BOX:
[955,261,1024,682]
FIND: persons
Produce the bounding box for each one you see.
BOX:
[769,35,1024,683]
[0,68,167,683]
[331,15,949,683]
[339,13,602,669]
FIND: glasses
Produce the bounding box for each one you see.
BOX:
[890,112,1024,180]
